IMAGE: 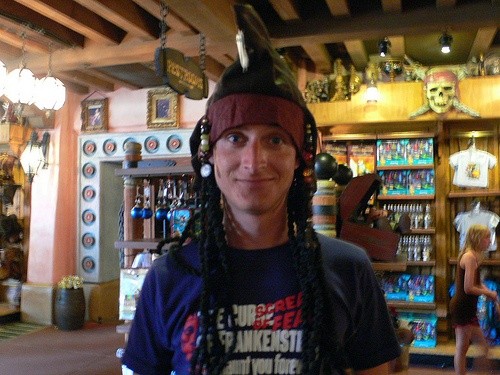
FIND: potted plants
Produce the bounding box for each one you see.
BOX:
[54,274,86,332]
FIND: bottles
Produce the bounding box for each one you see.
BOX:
[396,235,432,262]
[383,204,433,229]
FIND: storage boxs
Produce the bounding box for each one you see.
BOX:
[339,173,401,261]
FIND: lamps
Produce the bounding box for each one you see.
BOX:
[0,30,67,111]
[438,30,453,54]
[20,116,54,183]
[377,37,390,58]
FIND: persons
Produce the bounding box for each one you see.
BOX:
[121,4,403,375]
[449,225,497,375]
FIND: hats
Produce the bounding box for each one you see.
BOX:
[190,4,319,168]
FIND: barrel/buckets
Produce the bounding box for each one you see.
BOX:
[54,287,85,330]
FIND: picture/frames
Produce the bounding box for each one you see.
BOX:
[144,89,181,132]
[80,97,109,135]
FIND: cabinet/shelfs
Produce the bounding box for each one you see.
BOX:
[439,121,500,358]
[316,129,437,355]
